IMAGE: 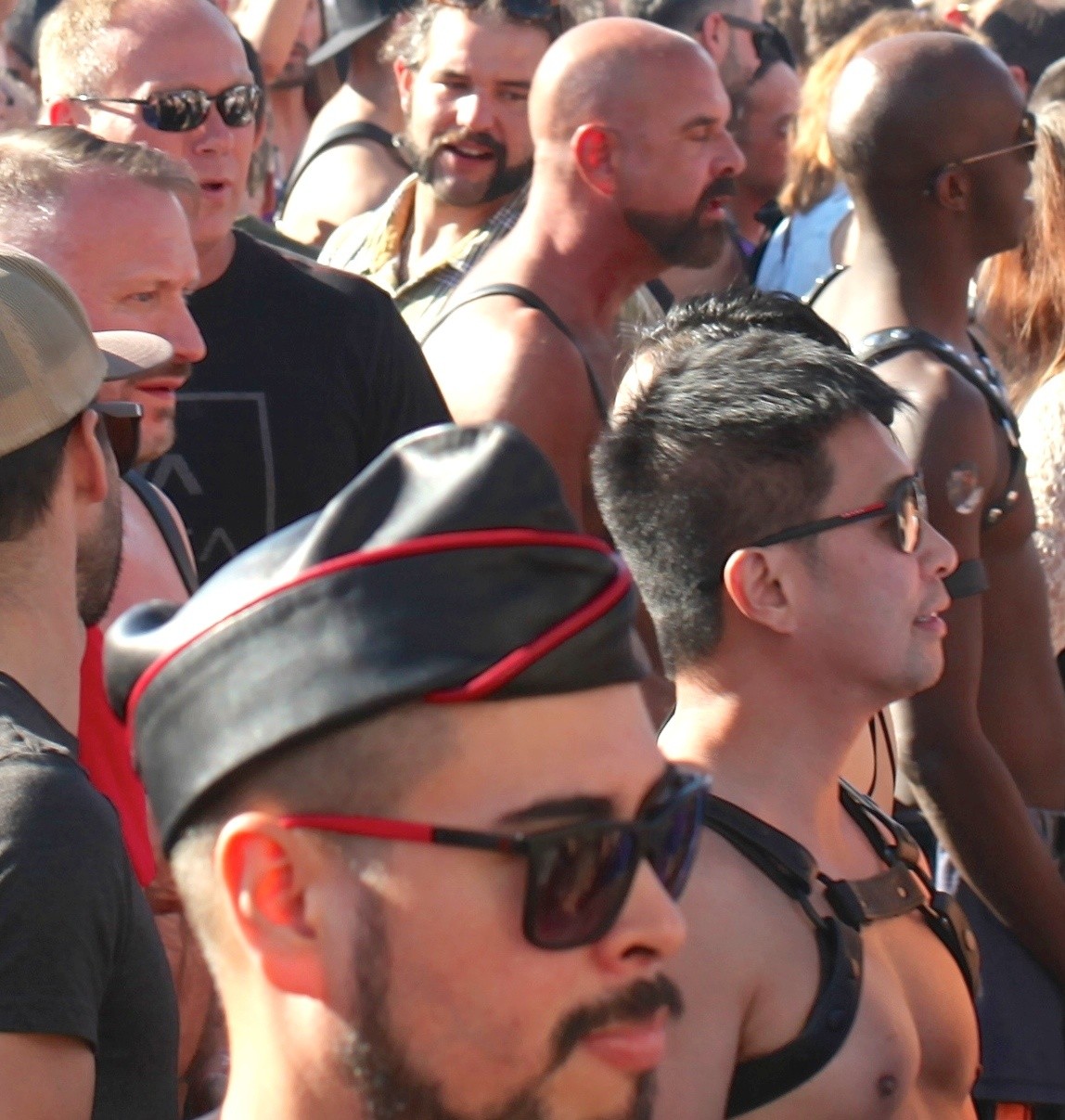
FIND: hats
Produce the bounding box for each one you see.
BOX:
[0,238,109,459]
[106,420,653,856]
[306,1,408,68]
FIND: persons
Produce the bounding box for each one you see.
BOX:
[32,1,463,590]
[0,124,234,1118]
[315,0,585,343]
[267,1,448,253]
[412,17,748,548]
[635,24,805,313]
[588,323,1001,1119]
[566,1,1064,146]
[0,0,69,129]
[227,31,456,431]
[790,28,1065,1120]
[970,92,1065,677]
[0,241,188,1116]
[214,0,345,186]
[754,12,973,299]
[103,418,714,1119]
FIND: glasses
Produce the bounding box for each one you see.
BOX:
[923,106,1041,199]
[76,398,143,478]
[696,13,776,54]
[272,763,707,949]
[70,82,265,135]
[722,473,923,577]
